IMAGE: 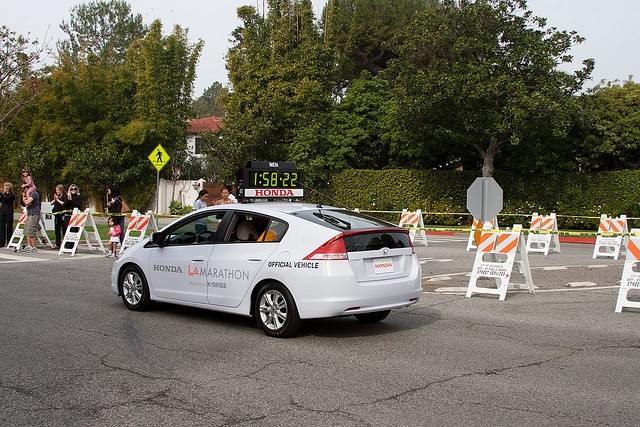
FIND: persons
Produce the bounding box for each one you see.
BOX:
[225,184,240,205]
[194,190,210,233]
[20,168,36,197]
[216,187,235,205]
[0,182,15,247]
[106,185,124,243]
[63,184,81,253]
[21,184,40,253]
[106,216,122,258]
[51,183,68,249]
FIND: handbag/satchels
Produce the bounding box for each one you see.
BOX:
[121,198,130,213]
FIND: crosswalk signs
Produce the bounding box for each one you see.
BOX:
[146,143,171,171]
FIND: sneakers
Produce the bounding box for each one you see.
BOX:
[22,246,38,252]
[106,252,115,258]
[52,246,59,248]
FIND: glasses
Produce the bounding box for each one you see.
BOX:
[70,188,77,191]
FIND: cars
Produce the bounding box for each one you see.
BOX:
[111,200,423,337]
